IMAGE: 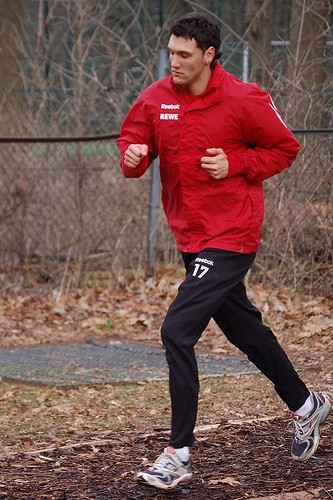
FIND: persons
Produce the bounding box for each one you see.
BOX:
[115,12,331,490]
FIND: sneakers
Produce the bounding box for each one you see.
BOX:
[287,391,332,461]
[137,445,192,492]
